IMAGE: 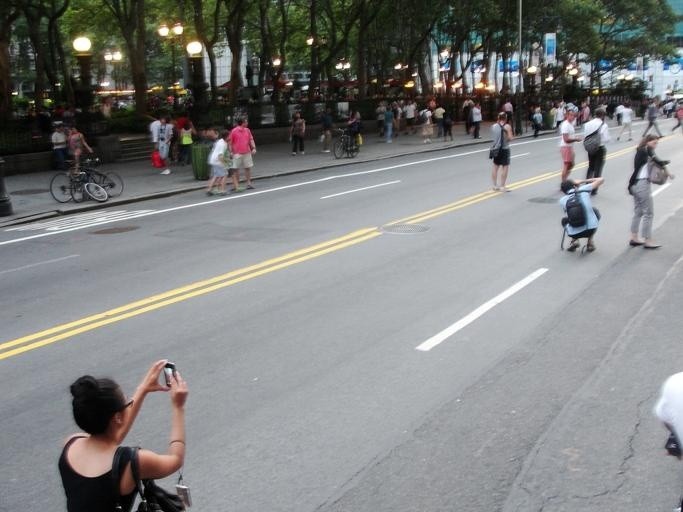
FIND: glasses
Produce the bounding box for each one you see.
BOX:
[125,398,134,409]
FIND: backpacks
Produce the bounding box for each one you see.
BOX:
[565,188,587,227]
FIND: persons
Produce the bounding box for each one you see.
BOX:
[148,93,683,178]
[51,125,67,169]
[558,108,587,186]
[582,106,609,196]
[55,356,189,511]
[556,177,605,252]
[626,134,674,249]
[67,127,91,168]
[655,362,682,512]
[490,110,513,191]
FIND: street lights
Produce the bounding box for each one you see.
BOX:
[266,32,583,132]
[71,21,212,156]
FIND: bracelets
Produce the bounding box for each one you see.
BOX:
[165,437,186,445]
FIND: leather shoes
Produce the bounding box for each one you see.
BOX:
[644,244,662,249]
[630,239,645,246]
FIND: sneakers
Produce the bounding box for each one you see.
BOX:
[493,185,511,193]
[206,185,254,196]
[586,243,595,251]
[568,240,580,251]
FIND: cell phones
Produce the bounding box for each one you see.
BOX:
[163,363,176,389]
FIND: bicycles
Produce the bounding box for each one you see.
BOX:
[330,127,363,160]
[49,153,126,205]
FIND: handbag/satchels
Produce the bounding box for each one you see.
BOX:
[648,163,668,184]
[152,151,166,168]
[420,112,428,122]
[111,446,185,512]
[584,130,600,154]
